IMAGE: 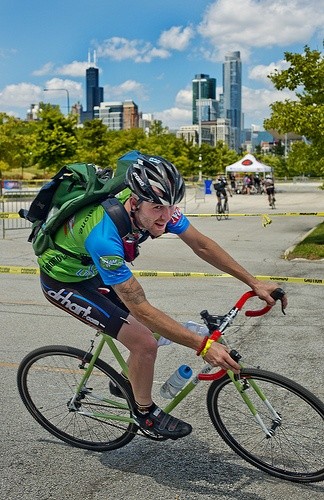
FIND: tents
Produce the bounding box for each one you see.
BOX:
[226,154,275,193]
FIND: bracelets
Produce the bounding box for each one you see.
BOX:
[196,335,214,359]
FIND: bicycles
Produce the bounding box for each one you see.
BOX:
[17,286,324,483]
[215,194,233,220]
[268,189,275,209]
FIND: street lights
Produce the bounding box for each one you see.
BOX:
[43,88,69,114]
[192,79,207,184]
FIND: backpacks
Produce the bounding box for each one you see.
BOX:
[17,159,134,267]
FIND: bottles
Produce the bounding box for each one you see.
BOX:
[160,365,193,399]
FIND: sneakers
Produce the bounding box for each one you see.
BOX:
[131,402,192,438]
[109,379,136,401]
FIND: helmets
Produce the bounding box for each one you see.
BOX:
[125,154,186,206]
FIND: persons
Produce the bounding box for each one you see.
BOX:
[38,154,287,439]
[216,173,276,213]
